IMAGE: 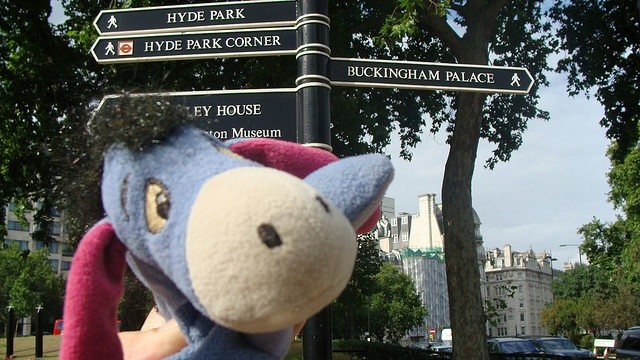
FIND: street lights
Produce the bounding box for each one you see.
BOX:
[545,255,557,280]
[559,244,582,264]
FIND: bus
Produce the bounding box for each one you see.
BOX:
[53,320,121,336]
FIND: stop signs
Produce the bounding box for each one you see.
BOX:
[430,328,436,334]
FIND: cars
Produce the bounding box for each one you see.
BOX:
[428,346,452,357]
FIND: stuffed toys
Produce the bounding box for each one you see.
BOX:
[56,96,393,359]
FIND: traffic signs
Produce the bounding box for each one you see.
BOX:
[294,50,535,94]
[91,20,331,64]
[87,1,333,36]
[87,81,332,151]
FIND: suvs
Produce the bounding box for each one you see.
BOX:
[488,338,562,360]
[526,337,597,360]
[616,326,640,360]
